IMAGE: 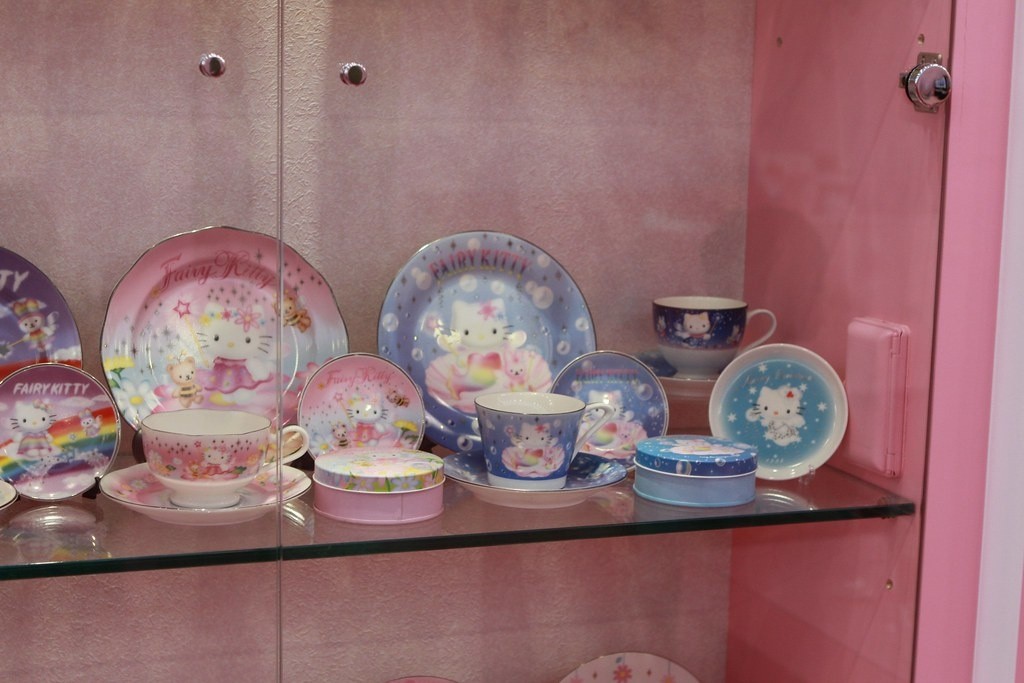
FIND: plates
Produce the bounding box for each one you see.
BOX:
[376,231,597,452]
[549,350,669,470]
[100,227,348,433]
[99,462,311,526]
[438,449,627,508]
[0,362,123,503]
[1,247,83,380]
[1,479,17,512]
[708,344,848,482]
[297,352,425,464]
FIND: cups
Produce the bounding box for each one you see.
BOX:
[652,296,777,379]
[475,391,614,491]
[140,408,309,509]
